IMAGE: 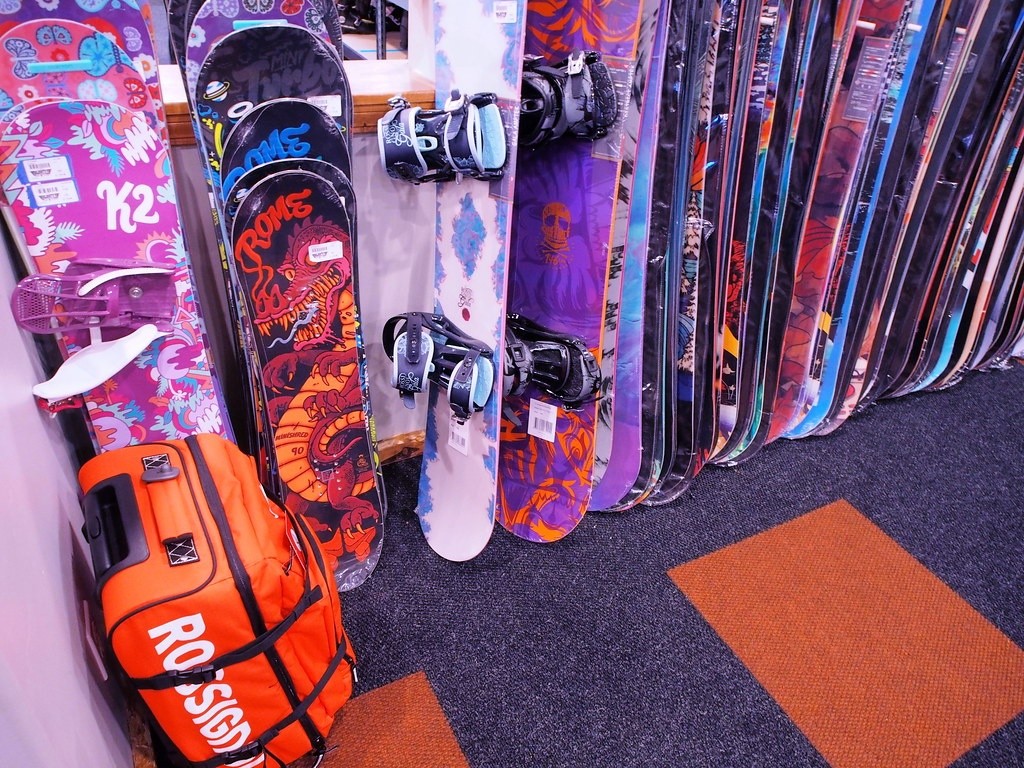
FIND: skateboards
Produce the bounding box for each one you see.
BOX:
[0,0,1024,600]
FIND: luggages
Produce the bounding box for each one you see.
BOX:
[78,434,360,768]
